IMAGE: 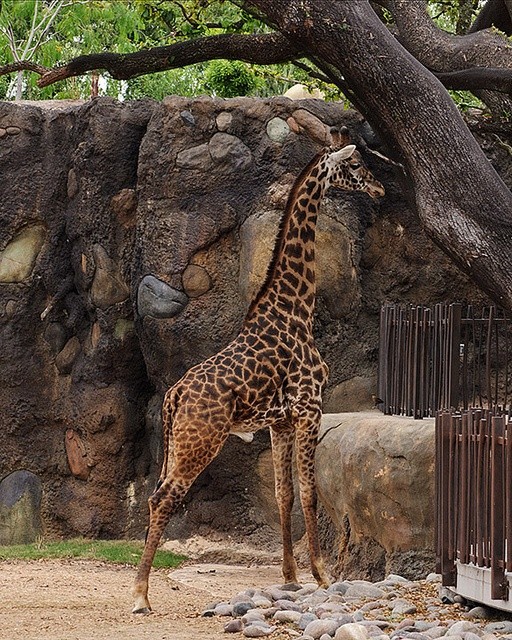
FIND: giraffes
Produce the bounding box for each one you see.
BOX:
[133,126,385,614]
